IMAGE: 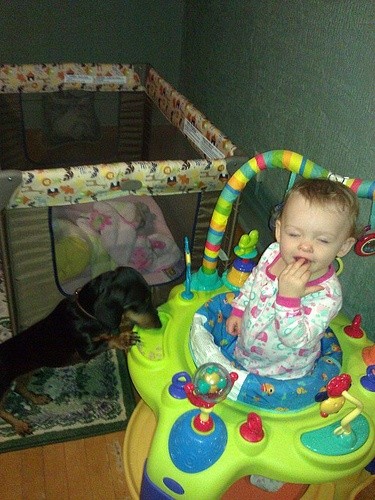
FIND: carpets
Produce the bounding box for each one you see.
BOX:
[0,338,138,454]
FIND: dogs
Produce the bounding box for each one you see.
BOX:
[0,266,163,439]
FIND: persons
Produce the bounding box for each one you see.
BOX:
[225,177,361,493]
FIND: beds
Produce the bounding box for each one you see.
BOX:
[0,61,250,340]
